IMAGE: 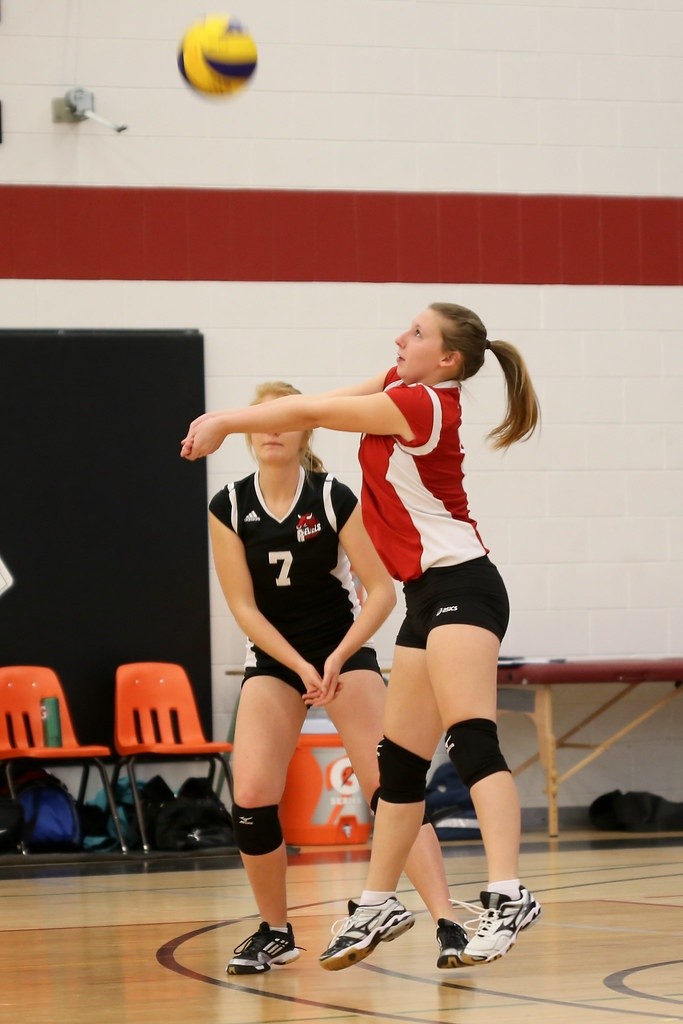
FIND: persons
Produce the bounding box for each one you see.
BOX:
[181,303,543,971]
[209,380,475,975]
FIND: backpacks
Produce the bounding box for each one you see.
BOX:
[8,772,239,854]
[422,761,487,843]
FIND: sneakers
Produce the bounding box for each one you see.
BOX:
[464,884,543,966]
[435,917,470,970]
[225,921,301,977]
[319,895,416,973]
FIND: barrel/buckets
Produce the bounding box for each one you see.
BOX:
[278,718,371,846]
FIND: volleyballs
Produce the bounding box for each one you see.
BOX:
[177,15,260,99]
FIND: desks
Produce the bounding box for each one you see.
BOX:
[496,656,683,838]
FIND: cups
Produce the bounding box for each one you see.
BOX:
[40,696,61,747]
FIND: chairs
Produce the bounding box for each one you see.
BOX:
[0,665,129,857]
[102,662,234,855]
